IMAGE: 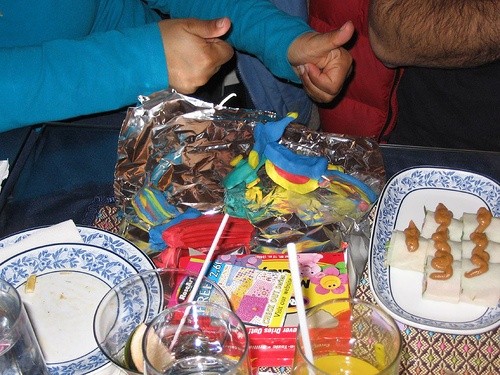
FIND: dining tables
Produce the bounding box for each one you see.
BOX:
[1,110,500,374]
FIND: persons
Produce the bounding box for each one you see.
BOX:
[305,0,500,152]
[0,0,359,237]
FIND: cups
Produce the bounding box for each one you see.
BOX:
[142,301,252,375]
[0,278,49,375]
[290,298,402,375]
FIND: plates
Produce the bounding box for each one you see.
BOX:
[0,243,150,375]
[0,225,165,375]
[367,165,500,336]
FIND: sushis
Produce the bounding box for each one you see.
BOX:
[420,200,500,307]
[384,219,428,272]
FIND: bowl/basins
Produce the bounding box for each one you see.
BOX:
[92,268,233,375]
